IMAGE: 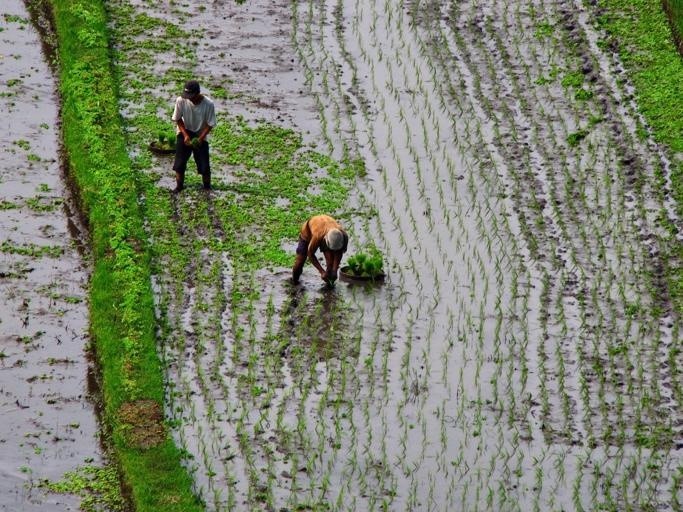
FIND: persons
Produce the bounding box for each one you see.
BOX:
[293,214,348,286]
[172,81,217,192]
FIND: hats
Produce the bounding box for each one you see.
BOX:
[181,80,200,99]
[326,229,344,250]
